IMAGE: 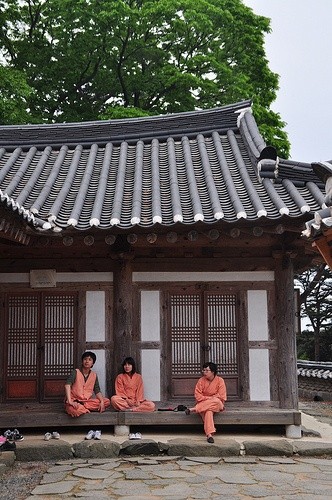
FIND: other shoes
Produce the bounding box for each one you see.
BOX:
[185,409,190,415]
[84,427,103,440]
[4,428,24,442]
[128,432,142,440]
[44,431,60,441]
[207,437,214,443]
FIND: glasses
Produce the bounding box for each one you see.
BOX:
[202,369,210,372]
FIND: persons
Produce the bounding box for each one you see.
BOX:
[64,352,111,417]
[185,362,227,443]
[111,357,155,412]
